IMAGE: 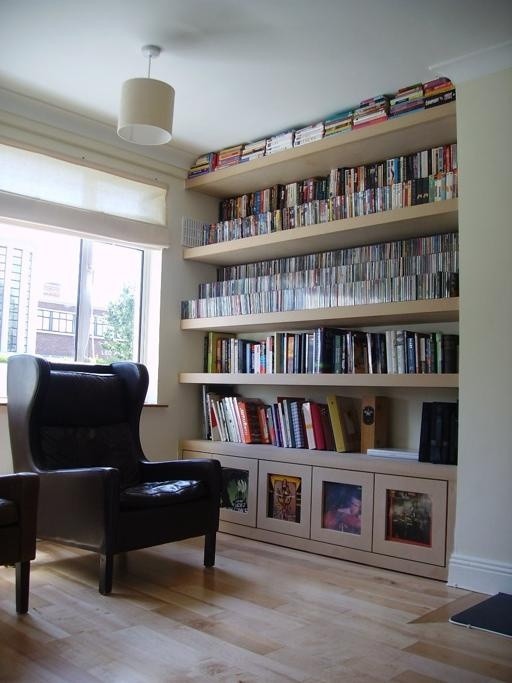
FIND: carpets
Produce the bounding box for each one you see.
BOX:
[448,591,512,638]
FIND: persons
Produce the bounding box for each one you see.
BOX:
[277,477,291,518]
[341,488,363,532]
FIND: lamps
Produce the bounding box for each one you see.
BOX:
[116,43,177,148]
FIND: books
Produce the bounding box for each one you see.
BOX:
[200,143,459,246]
[202,385,459,464]
[188,76,456,179]
[186,232,459,319]
[204,327,460,375]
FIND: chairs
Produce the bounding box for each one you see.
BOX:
[6,354,223,595]
[0,471,40,612]
